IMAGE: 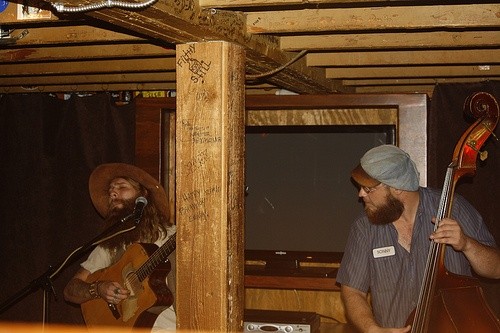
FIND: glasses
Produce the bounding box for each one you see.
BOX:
[363,184,386,193]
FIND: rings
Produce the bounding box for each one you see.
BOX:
[114,287,120,294]
[112,297,117,303]
[114,293,118,299]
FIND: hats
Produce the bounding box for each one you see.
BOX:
[351,144,420,191]
[89,162,170,223]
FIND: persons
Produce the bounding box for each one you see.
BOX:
[336,144,500,333]
[63,163,177,333]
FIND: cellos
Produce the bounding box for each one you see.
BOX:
[405,90,500,333]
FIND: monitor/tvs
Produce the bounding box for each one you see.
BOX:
[245,124,397,276]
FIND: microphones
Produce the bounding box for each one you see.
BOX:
[133,196,147,224]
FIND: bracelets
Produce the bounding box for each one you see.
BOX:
[89,281,99,298]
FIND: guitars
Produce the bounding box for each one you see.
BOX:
[80,186,249,329]
